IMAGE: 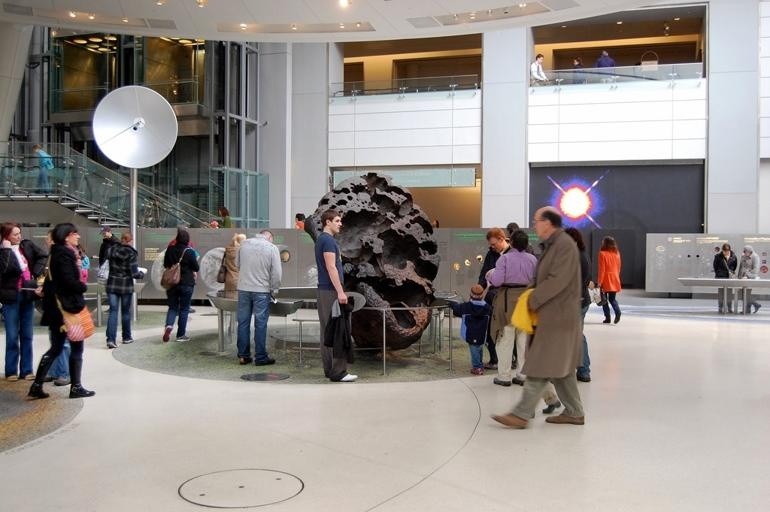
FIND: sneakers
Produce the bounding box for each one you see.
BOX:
[576,372,591,382]
[240,356,252,365]
[512,377,526,386]
[107,339,118,348]
[176,335,190,341]
[123,337,134,344]
[54,376,71,385]
[493,377,512,386]
[483,363,496,370]
[24,373,36,380]
[7,374,18,381]
[43,375,52,382]
[511,363,518,369]
[163,325,173,342]
[471,368,484,375]
[255,358,275,366]
[339,374,358,382]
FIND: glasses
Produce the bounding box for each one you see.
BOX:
[532,219,546,225]
[68,233,79,237]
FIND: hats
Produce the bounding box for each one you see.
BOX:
[470,285,485,300]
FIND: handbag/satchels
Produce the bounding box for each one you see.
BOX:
[718,253,736,279]
[20,273,40,302]
[96,248,111,285]
[597,286,607,306]
[48,254,96,342]
[217,252,227,283]
[161,248,192,288]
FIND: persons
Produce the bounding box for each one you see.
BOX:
[96,226,120,306]
[596,236,622,323]
[486,231,538,386]
[564,227,595,382]
[714,242,738,313]
[211,206,232,227]
[531,55,554,86]
[454,284,492,375]
[480,226,511,283]
[572,58,586,85]
[31,143,53,193]
[315,209,359,381]
[30,222,96,399]
[740,245,761,313]
[218,233,247,355]
[0,220,49,382]
[294,212,306,228]
[633,61,645,82]
[595,49,619,83]
[491,205,584,429]
[233,231,282,365]
[159,231,200,341]
[105,232,146,348]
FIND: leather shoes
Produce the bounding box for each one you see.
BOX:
[728,307,733,313]
[740,308,751,314]
[490,413,528,429]
[546,414,585,425]
[719,307,722,314]
[753,303,761,313]
[603,315,611,323]
[70,384,95,398]
[614,312,621,324]
[28,385,50,398]
[543,401,561,413]
